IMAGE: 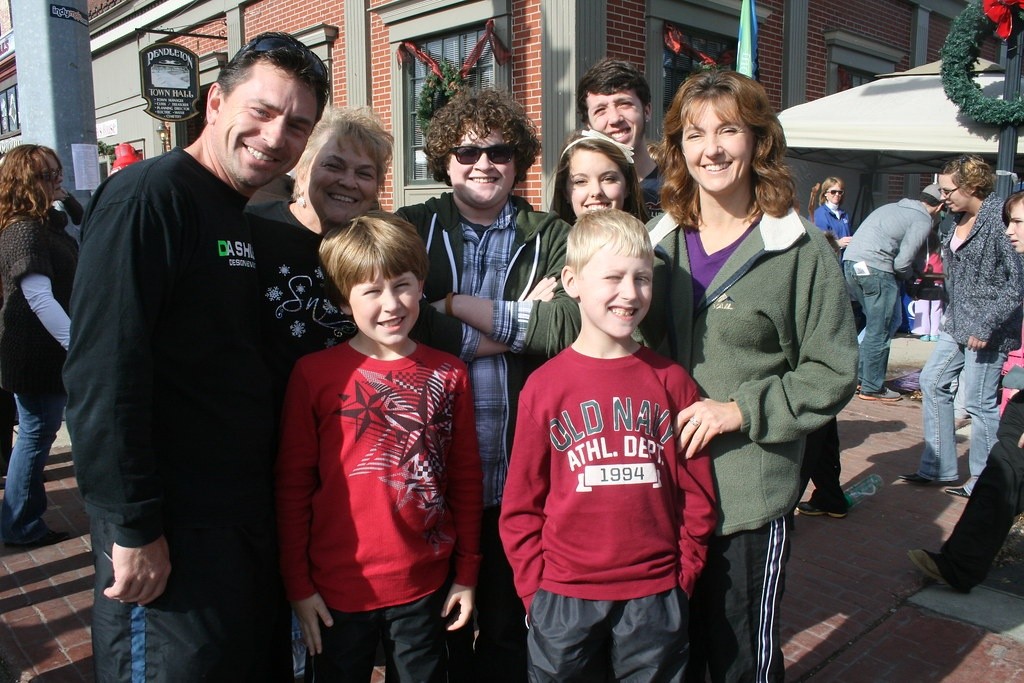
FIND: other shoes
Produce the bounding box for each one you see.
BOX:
[907,547,971,596]
[10,527,73,550]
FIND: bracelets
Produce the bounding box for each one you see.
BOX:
[445,291,458,317]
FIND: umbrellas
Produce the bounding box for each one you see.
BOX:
[736,0,759,80]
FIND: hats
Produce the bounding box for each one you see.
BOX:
[922,183,942,201]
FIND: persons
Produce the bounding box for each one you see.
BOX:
[0,30,1024,682]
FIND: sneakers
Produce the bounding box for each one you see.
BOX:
[855,382,902,402]
[796,497,847,518]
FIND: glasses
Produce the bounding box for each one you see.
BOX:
[937,188,959,196]
[826,190,844,196]
[217,38,328,83]
[32,167,64,180]
[449,144,518,166]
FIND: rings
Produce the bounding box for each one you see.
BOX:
[689,417,701,427]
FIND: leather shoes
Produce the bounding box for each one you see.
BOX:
[898,472,932,483]
[945,487,969,498]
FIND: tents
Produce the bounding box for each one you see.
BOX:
[775,55,1024,162]
